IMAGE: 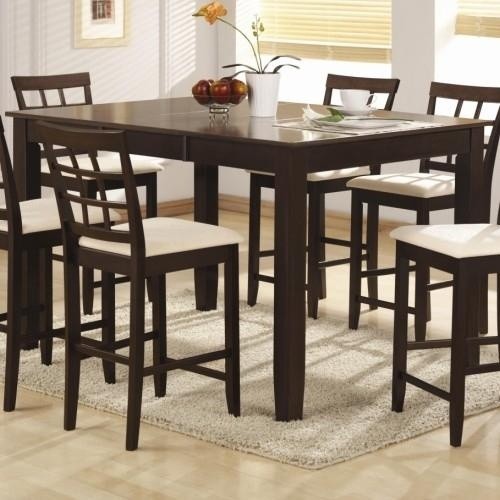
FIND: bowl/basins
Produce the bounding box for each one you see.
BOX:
[192,94,247,115]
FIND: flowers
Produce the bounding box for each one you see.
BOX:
[190,1,300,81]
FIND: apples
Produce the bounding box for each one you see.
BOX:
[212,81,231,103]
[231,79,247,105]
[191,80,211,104]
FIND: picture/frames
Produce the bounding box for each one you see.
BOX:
[72,0,131,49]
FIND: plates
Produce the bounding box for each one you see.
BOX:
[332,107,375,116]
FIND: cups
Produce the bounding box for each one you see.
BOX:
[340,89,377,111]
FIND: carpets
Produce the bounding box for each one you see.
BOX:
[0,288,499,472]
